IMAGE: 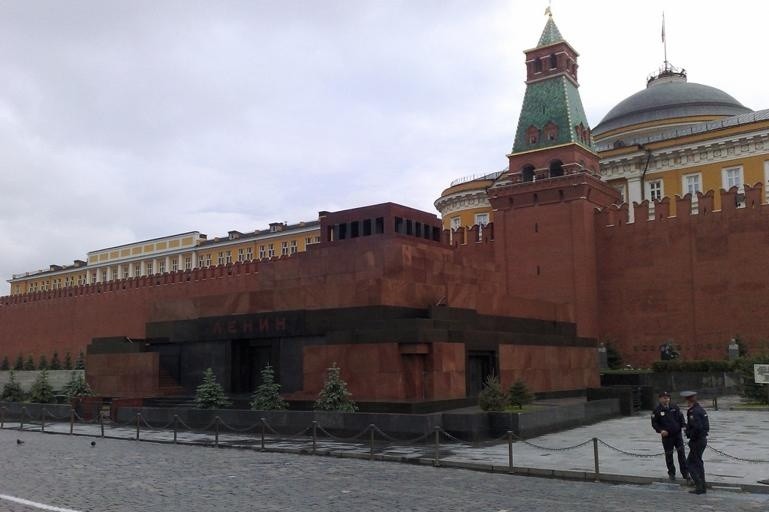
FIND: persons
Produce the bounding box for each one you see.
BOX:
[679,391,710,495]
[658,343,681,359]
[650,391,691,480]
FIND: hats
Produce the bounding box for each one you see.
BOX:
[679,390,697,399]
[659,391,670,397]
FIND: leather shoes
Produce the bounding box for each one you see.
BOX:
[689,489,705,495]
